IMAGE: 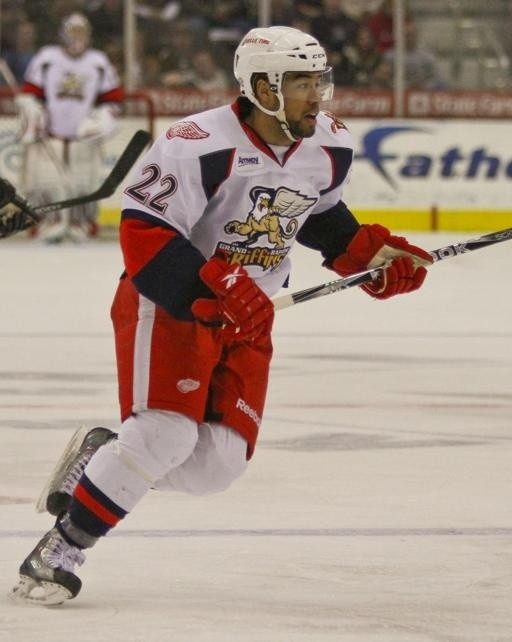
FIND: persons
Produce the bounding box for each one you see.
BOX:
[11,24,432,599]
[0,0,258,92]
[272,0,438,91]
[13,11,128,244]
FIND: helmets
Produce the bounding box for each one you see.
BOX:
[233,25,327,116]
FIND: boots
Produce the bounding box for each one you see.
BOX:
[20,424,118,599]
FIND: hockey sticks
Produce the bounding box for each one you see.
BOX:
[33,129,152,218]
[1,61,69,243]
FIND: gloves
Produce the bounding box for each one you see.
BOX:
[321,224,435,300]
[190,255,274,345]
